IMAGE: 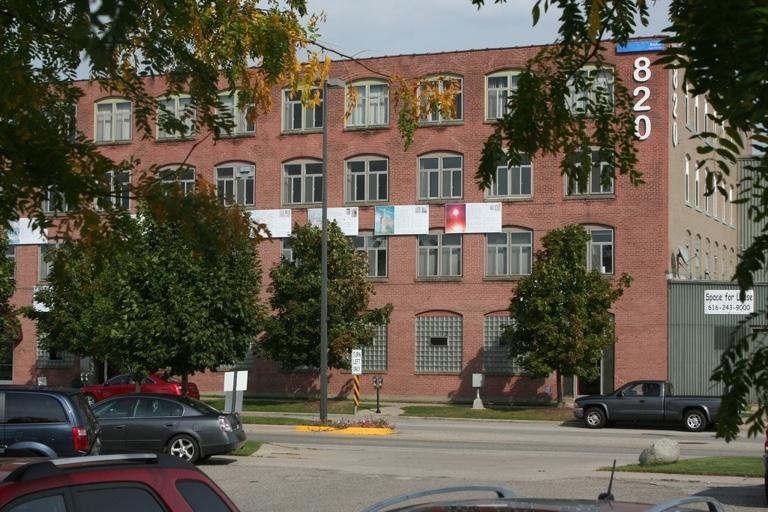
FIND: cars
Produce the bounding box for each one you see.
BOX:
[349,456,744,510]
[1,371,250,512]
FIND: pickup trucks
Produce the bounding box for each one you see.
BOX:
[571,379,722,433]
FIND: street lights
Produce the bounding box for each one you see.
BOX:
[312,73,349,429]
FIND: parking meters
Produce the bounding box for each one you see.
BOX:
[373,376,385,415]
